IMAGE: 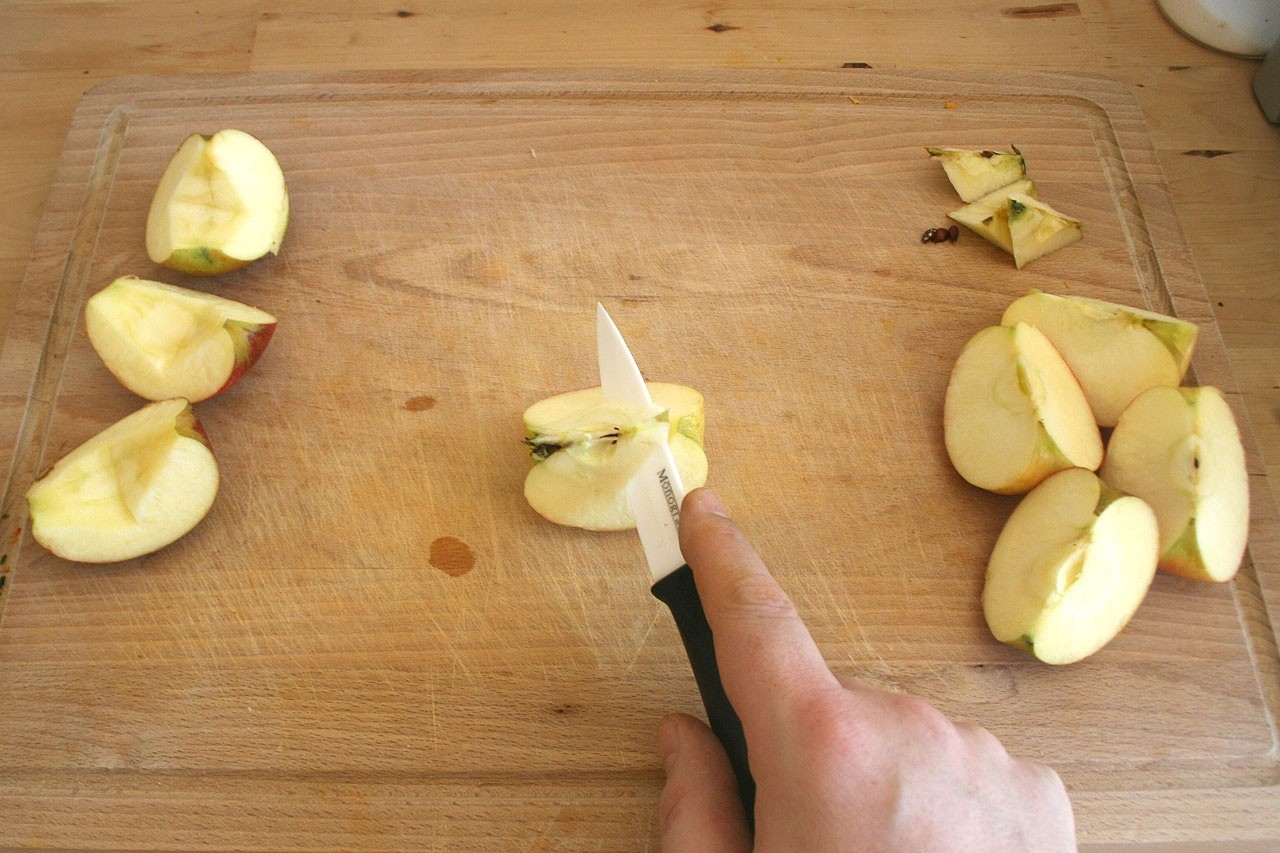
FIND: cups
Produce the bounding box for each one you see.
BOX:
[1157,0,1279,59]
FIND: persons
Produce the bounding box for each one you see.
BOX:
[658,487,1076,853]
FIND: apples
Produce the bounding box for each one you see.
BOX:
[85,276,277,405]
[983,467,1161,665]
[1096,387,1250,582]
[24,398,221,563]
[145,128,289,276]
[942,320,1105,494]
[999,291,1198,427]
[521,381,710,533]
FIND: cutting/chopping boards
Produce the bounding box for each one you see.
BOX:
[0,75,1279,852]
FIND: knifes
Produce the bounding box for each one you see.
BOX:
[596,303,760,846]
